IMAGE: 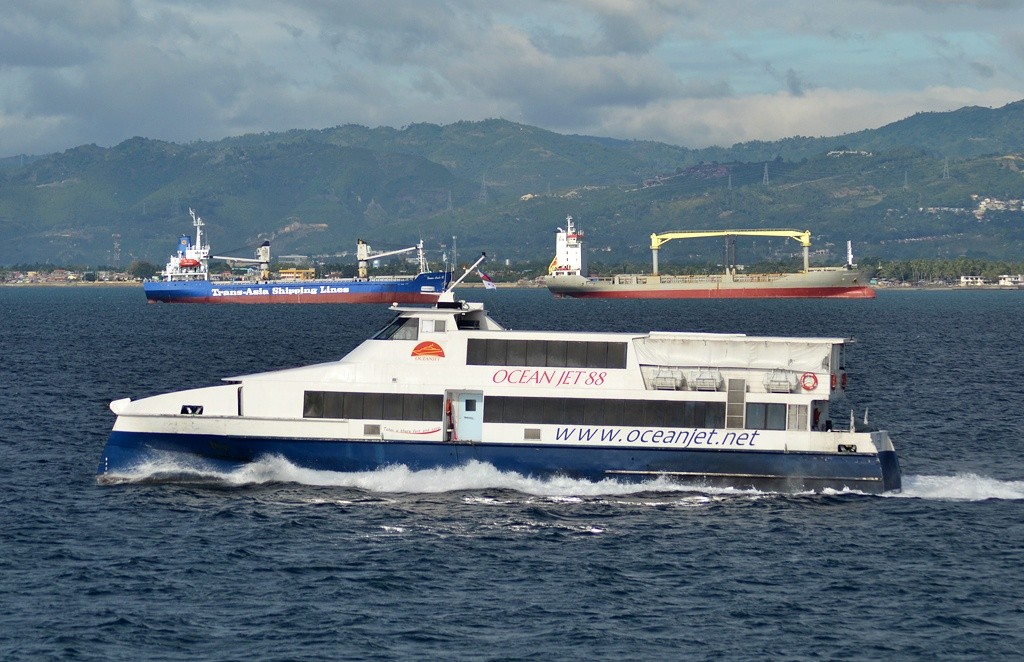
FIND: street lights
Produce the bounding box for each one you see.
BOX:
[452,235,457,282]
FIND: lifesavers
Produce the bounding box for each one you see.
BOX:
[814,408,819,424]
[800,372,818,390]
[446,399,451,416]
[832,374,836,390]
[841,372,846,389]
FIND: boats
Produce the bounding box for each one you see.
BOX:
[142,207,455,305]
[545,215,877,299]
[95,250,903,499]
